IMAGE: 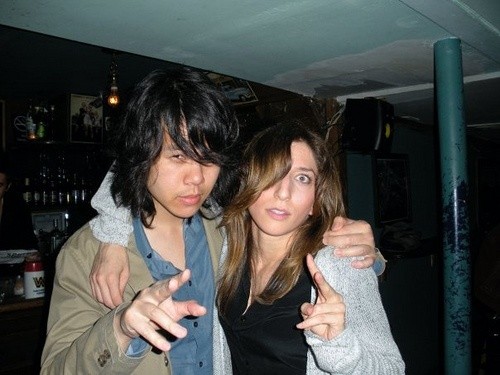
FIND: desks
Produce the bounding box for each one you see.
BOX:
[0,298,49,375]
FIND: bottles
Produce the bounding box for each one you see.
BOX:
[47,214,64,254]
[37,157,51,203]
[19,162,33,201]
[55,155,67,202]
[25,99,37,140]
[75,166,87,204]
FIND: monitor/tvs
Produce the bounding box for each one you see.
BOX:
[372,152,412,228]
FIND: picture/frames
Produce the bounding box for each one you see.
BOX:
[69,93,105,145]
[371,152,412,228]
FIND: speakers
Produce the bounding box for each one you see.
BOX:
[342,98,395,155]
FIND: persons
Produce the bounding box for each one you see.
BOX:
[90,113,404,375]
[1,172,37,250]
[41,70,387,375]
